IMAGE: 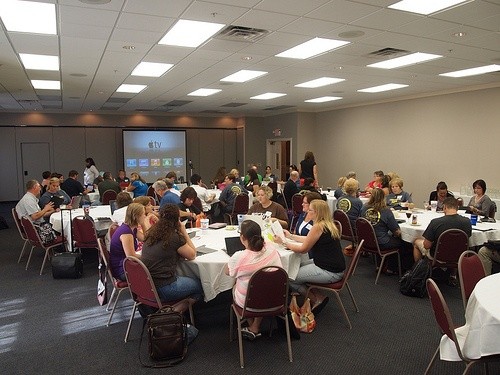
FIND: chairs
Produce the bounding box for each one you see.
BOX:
[11,184,488,375]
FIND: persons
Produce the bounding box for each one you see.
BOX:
[110,187,347,337]
[413,196,472,265]
[16,170,148,247]
[466,179,491,217]
[299,177,327,201]
[334,170,415,274]
[84,157,99,185]
[427,181,454,210]
[299,151,319,190]
[153,165,299,230]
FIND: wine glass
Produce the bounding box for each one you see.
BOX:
[406,211,412,224]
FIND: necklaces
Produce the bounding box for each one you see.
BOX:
[475,195,484,204]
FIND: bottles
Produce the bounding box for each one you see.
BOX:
[457,198,462,210]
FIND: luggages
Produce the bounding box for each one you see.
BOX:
[51,209,84,280]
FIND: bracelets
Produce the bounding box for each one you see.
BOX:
[473,208,477,213]
[288,234,292,239]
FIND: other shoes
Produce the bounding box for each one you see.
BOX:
[312,296,330,319]
[376,266,397,274]
[238,328,261,340]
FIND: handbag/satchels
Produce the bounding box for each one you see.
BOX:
[290,296,316,333]
[484,239,500,251]
[96,245,109,305]
[137,312,188,368]
[343,244,368,257]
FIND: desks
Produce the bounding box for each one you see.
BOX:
[392,207,500,248]
[238,181,286,192]
[179,189,253,209]
[319,190,370,217]
[134,226,302,322]
[79,189,134,201]
[437,271,500,361]
[49,205,112,232]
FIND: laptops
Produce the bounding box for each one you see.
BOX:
[225,237,246,257]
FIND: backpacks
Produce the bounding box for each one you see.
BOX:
[399,255,432,298]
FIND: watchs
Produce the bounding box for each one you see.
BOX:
[282,241,288,247]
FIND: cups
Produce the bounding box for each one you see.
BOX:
[211,184,214,189]
[241,176,245,181]
[236,182,239,184]
[431,201,437,212]
[83,205,89,216]
[412,215,417,226]
[471,213,477,221]
[200,218,209,230]
[327,187,331,192]
